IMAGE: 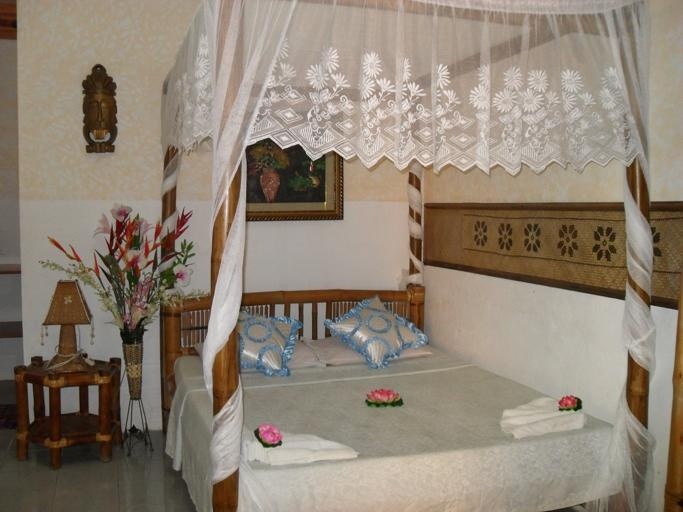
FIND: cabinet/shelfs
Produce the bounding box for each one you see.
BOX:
[14,356,123,469]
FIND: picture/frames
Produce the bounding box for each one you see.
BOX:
[241,83,347,222]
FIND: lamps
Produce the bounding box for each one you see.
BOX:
[38,277,93,373]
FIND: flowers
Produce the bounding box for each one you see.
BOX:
[557,393,582,412]
[255,422,282,446]
[364,387,404,408]
[36,202,198,343]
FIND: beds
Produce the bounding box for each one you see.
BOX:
[161,1,656,512]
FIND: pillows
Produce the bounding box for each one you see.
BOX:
[191,336,325,375]
[218,307,303,385]
[306,335,433,366]
[324,294,427,369]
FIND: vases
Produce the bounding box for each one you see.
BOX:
[122,345,159,451]
